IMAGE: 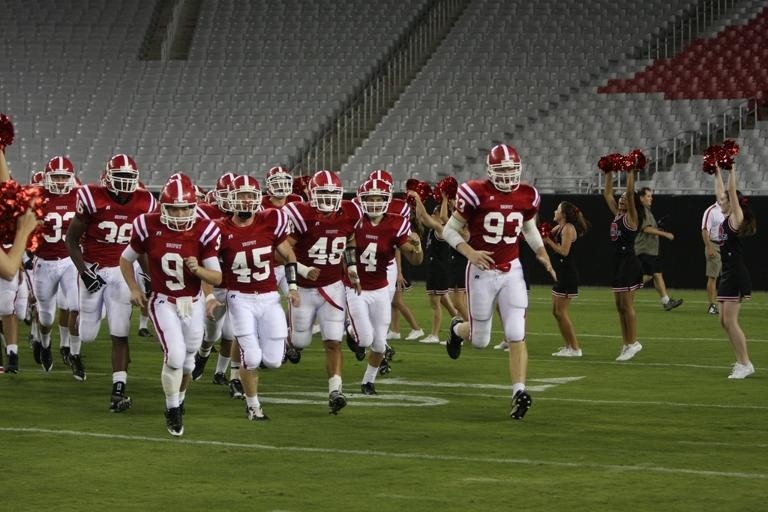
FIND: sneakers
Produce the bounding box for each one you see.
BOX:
[32,340,41,365]
[40,339,54,372]
[164,406,184,437]
[68,353,88,382]
[178,399,187,416]
[492,297,756,380]
[246,403,270,422]
[260,324,401,376]
[4,362,19,375]
[361,382,377,395]
[446,314,465,360]
[227,378,246,400]
[328,390,349,413]
[59,345,71,366]
[108,392,134,414]
[192,345,227,386]
[404,327,463,350]
[138,327,154,338]
[508,391,532,420]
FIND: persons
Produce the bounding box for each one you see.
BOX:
[603,155,647,361]
[443,144,558,421]
[634,187,683,311]
[709,151,757,379]
[540,201,587,357]
[493,232,530,351]
[702,200,726,314]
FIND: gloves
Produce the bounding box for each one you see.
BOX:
[79,269,102,294]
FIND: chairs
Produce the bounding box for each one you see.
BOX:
[0,0,432,187]
[335,1,768,194]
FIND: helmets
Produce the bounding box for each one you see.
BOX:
[43,155,76,195]
[310,170,344,213]
[166,172,192,187]
[205,190,218,206]
[159,179,197,232]
[486,143,522,193]
[215,172,240,214]
[31,171,45,186]
[226,175,262,219]
[193,185,207,204]
[368,170,393,183]
[104,153,139,193]
[266,164,294,199]
[355,178,393,217]
